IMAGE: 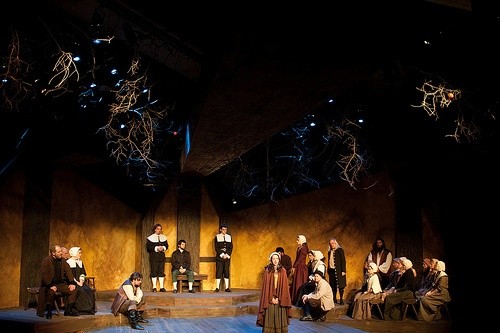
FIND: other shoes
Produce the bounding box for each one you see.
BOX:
[225,288,231,292]
[340,299,344,305]
[160,288,166,292]
[173,289,178,293]
[315,316,326,322]
[299,315,313,321]
[189,289,195,293]
[334,300,337,303]
[153,288,157,292]
[215,288,219,292]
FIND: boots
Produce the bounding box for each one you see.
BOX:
[64,302,79,316]
[46,304,52,319]
[128,309,144,330]
[135,310,149,323]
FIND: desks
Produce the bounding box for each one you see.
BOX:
[85,277,96,290]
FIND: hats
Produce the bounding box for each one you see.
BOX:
[313,251,324,261]
[368,263,378,272]
[423,259,430,267]
[298,235,306,246]
[393,258,400,263]
[268,252,281,264]
[69,247,81,257]
[436,261,445,272]
[400,257,413,270]
[313,270,326,279]
[432,259,439,265]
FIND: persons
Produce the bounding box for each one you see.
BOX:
[147,223,169,292]
[37,245,95,319]
[111,272,148,330]
[327,238,346,305]
[171,239,194,293]
[214,225,233,292]
[300,270,335,322]
[292,235,308,304]
[256,252,292,333]
[295,249,326,306]
[348,238,451,321]
[276,247,292,277]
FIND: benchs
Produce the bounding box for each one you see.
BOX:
[25,287,96,319]
[175,273,209,292]
[370,296,449,322]
